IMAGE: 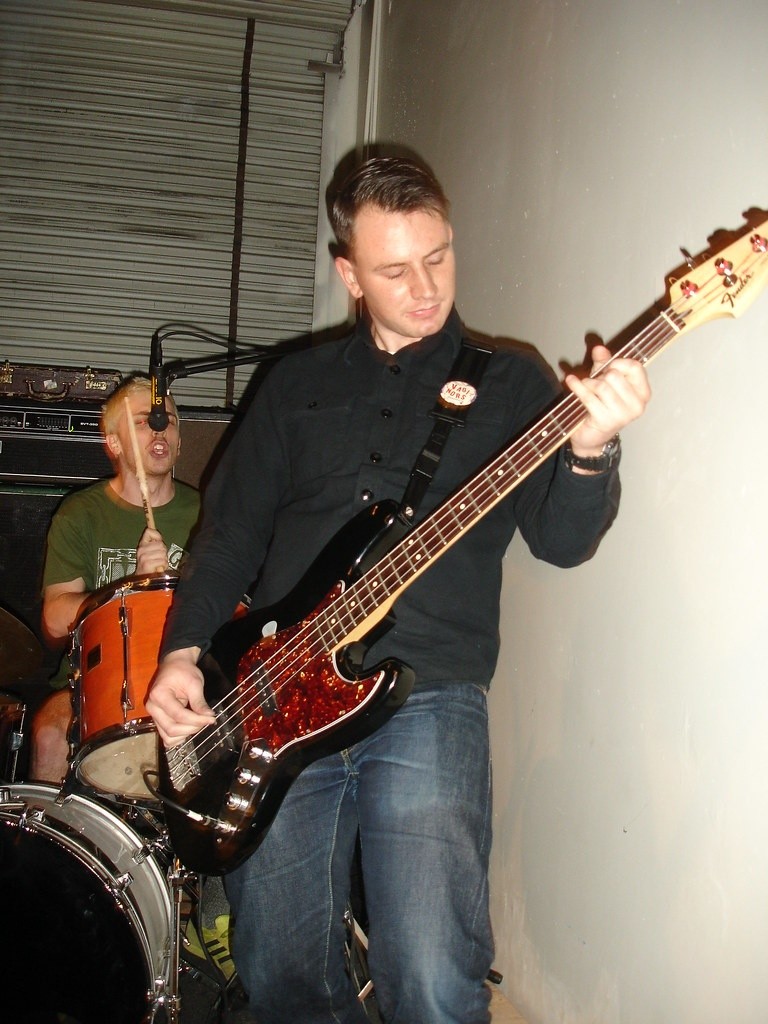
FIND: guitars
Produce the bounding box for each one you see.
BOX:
[156,216,768,874]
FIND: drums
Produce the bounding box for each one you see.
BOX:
[67,569,181,815]
[1,781,182,1024]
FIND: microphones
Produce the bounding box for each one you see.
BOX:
[146,335,169,432]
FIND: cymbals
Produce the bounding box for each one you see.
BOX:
[0,607,42,729]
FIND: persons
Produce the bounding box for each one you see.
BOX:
[31,378,205,784]
[144,153,650,1024]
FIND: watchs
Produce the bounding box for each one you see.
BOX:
[564,437,621,472]
